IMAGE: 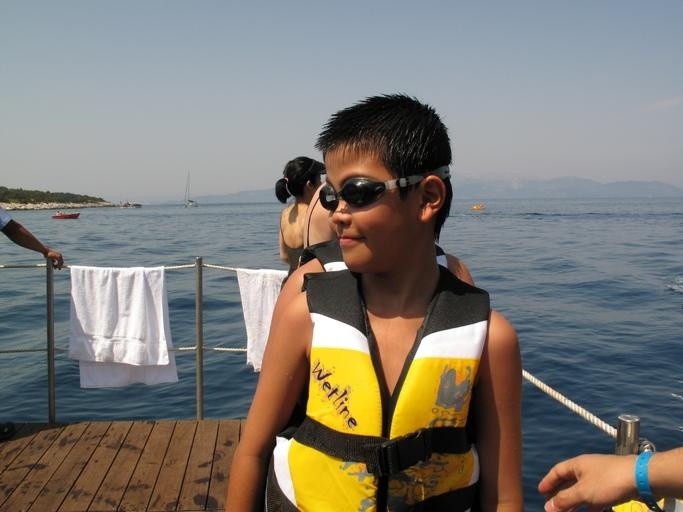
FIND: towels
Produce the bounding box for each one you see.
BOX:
[68,266,179,390]
[236,266,289,373]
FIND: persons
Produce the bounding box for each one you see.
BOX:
[537,446,682,512]
[273,154,325,274]
[300,179,338,248]
[0,202,64,271]
[222,92,524,511]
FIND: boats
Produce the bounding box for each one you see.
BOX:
[184,170,196,207]
[120,200,142,208]
[471,203,486,210]
[51,211,80,219]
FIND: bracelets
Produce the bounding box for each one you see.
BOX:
[632,451,658,507]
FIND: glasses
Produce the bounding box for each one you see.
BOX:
[317,166,452,212]
